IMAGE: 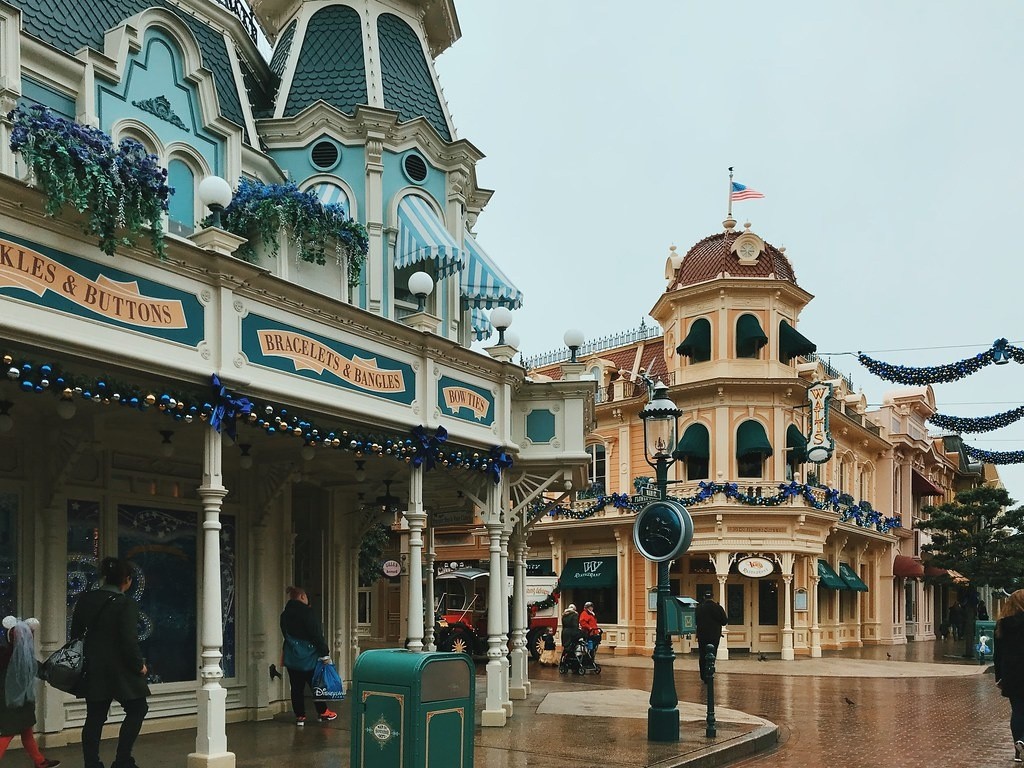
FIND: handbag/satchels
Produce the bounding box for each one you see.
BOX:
[311,660,344,701]
[283,638,317,671]
[40,636,86,695]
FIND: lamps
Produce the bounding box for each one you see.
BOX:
[301,440,316,459]
[198,176,232,230]
[58,397,79,420]
[223,423,252,470]
[564,329,584,363]
[0,400,15,432]
[457,490,466,507]
[161,430,176,456]
[408,272,433,312]
[490,306,513,344]
[503,333,519,362]
[354,460,367,481]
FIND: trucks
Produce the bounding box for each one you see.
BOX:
[404,568,560,661]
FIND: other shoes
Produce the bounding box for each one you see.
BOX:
[36,758,60,768]
[1015,741,1024,761]
[1013,753,1022,761]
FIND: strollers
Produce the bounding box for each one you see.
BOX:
[559,627,603,676]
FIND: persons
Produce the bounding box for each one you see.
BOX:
[940,601,990,641]
[0,616,61,768]
[71,557,151,768]
[993,589,1024,761]
[280,587,338,726]
[696,590,728,683]
[540,601,599,673]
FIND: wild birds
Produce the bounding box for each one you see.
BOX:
[843,697,854,705]
[886,652,891,658]
[758,655,767,661]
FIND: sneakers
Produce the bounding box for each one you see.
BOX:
[295,716,308,725]
[316,709,336,723]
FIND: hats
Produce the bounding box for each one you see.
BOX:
[704,591,714,597]
[584,602,593,608]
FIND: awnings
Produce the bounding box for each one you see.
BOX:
[893,554,970,587]
[559,556,617,589]
[839,563,868,592]
[818,559,848,590]
[462,230,523,341]
[393,194,467,280]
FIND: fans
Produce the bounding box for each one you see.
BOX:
[362,480,401,510]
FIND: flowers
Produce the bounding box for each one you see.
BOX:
[7,101,169,262]
[199,172,368,288]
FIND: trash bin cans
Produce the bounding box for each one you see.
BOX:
[350,648,476,768]
[974,620,997,660]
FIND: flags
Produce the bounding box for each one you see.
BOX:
[731,181,764,200]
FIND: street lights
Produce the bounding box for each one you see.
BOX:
[638,375,683,744]
